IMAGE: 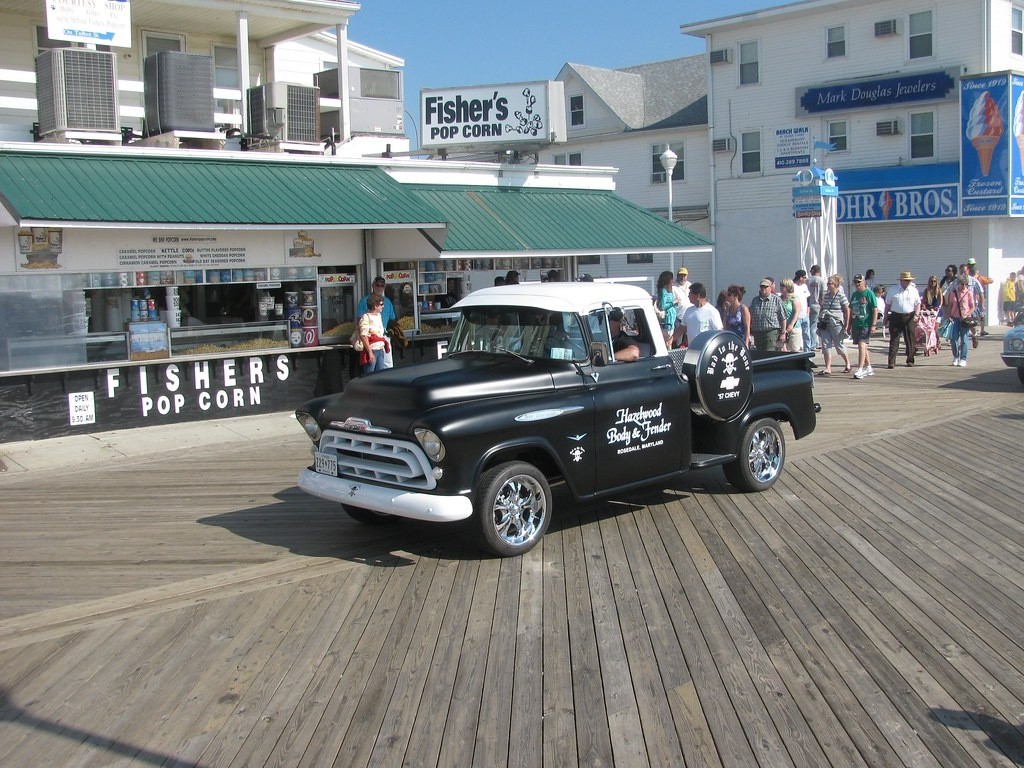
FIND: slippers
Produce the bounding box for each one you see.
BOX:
[818,369,832,375]
[842,366,853,373]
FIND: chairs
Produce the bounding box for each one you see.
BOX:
[536,338,656,359]
[877,312,890,339]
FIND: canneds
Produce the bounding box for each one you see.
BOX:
[131,299,155,322]
[428,300,433,310]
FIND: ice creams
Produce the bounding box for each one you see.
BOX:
[966,90,1003,177]
[1013,90,1024,176]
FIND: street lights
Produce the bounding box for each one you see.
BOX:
[659,143,680,277]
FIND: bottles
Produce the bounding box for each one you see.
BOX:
[429,301,432,309]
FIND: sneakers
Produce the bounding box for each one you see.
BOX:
[952,358,959,366]
[960,358,967,366]
[854,370,864,379]
[862,366,875,377]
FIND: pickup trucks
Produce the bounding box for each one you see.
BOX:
[295,280,822,559]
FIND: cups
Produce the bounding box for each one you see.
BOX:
[259,297,283,319]
[294,232,313,256]
[31,227,49,250]
[418,301,441,309]
[48,231,62,253]
[18,235,33,254]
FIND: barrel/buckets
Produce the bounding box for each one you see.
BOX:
[458,260,472,271]
[232,269,266,282]
[419,274,446,294]
[71,291,92,334]
[206,270,231,283]
[285,291,319,348]
[475,259,492,270]
[62,274,114,288]
[131,300,159,322]
[136,272,174,286]
[165,287,182,328]
[118,273,128,286]
[531,257,562,270]
[176,271,203,284]
[496,258,529,270]
[271,268,316,279]
[419,261,456,271]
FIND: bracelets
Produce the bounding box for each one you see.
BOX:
[781,330,786,334]
[914,314,917,316]
[871,324,877,326]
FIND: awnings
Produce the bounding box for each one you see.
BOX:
[0,152,450,249]
[400,182,715,258]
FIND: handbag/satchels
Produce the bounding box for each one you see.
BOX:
[349,311,370,352]
[962,318,978,327]
[653,299,666,320]
[817,318,827,330]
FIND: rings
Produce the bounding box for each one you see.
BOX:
[787,332,788,333]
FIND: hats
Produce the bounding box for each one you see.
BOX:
[852,274,865,282]
[896,271,916,280]
[676,267,689,275]
[966,258,977,265]
[759,279,772,287]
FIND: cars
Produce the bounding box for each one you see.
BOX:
[1000,324,1024,384]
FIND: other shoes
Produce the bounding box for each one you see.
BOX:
[888,363,895,369]
[907,361,914,367]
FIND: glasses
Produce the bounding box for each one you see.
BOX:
[374,284,385,287]
[378,304,383,306]
[854,281,861,284]
[931,281,936,282]
[960,282,968,286]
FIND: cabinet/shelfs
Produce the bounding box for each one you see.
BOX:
[417,256,563,295]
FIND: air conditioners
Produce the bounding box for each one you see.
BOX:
[35,47,122,141]
[710,48,732,66]
[874,19,901,38]
[246,81,321,155]
[713,138,734,153]
[142,50,215,132]
[876,118,903,137]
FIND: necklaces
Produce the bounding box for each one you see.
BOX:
[676,282,687,292]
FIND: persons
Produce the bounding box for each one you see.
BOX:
[792,269,812,363]
[818,274,852,375]
[547,270,559,282]
[716,285,751,351]
[1003,266,1024,324]
[671,267,695,349]
[848,273,878,379]
[749,277,803,352]
[355,276,397,368]
[435,278,458,308]
[668,282,724,347]
[556,307,640,362]
[358,293,390,374]
[805,265,825,352]
[866,256,994,369]
[495,271,521,286]
[580,274,593,282]
[656,270,679,350]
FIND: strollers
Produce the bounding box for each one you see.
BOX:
[914,307,938,356]
